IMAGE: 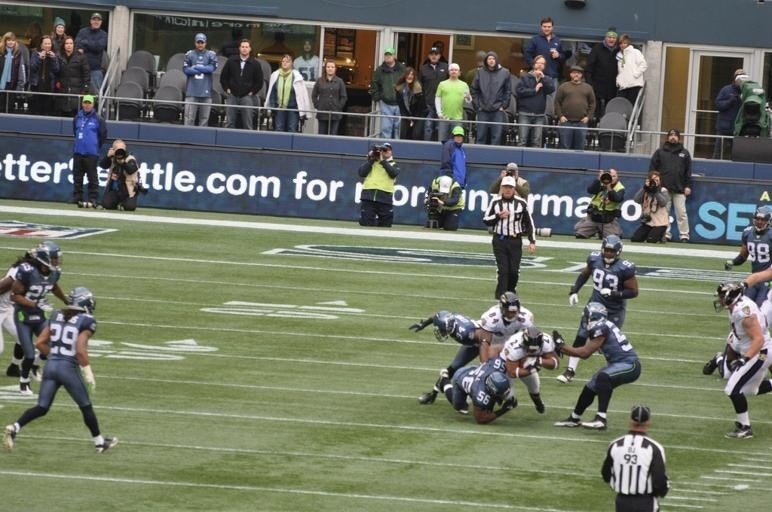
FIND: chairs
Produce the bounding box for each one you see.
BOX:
[114,50,271,129]
[463,74,633,152]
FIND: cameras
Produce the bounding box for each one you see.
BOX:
[648,178,657,189]
[506,170,512,177]
[115,148,126,159]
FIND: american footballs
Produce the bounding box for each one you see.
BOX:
[519,359,527,368]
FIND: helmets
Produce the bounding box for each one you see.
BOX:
[600,234,623,264]
[521,326,544,355]
[431,310,458,343]
[36,241,65,271]
[714,279,744,313]
[65,286,97,314]
[581,301,608,331]
[498,291,521,322]
[751,207,772,234]
[486,370,512,400]
[28,248,36,259]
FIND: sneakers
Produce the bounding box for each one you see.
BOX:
[576,418,608,430]
[552,417,581,428]
[5,367,23,376]
[681,239,688,243]
[95,437,120,454]
[703,352,725,375]
[19,387,34,396]
[555,367,576,385]
[76,199,96,209]
[432,368,449,392]
[723,428,753,441]
[418,392,436,404]
[502,396,514,410]
[659,237,669,244]
[533,399,545,416]
[2,422,19,454]
[457,408,471,417]
[31,370,42,383]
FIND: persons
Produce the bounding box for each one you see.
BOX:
[357,142,401,227]
[370,16,649,151]
[10,241,67,396]
[99,140,141,211]
[70,95,108,208]
[409,233,641,430]
[220,39,346,140]
[0,13,107,118]
[182,33,218,126]
[573,129,692,244]
[425,126,535,300]
[599,402,669,511]
[713,69,772,160]
[3,286,119,454]
[1,247,43,382]
[702,204,771,439]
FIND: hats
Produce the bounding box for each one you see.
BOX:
[382,143,393,151]
[91,13,102,21]
[438,176,452,193]
[54,16,65,30]
[570,65,583,73]
[429,46,440,56]
[606,26,618,38]
[195,33,207,44]
[452,125,465,136]
[735,68,746,76]
[629,404,652,424]
[449,62,460,71]
[82,94,96,105]
[667,129,680,138]
[384,47,395,55]
[501,163,518,187]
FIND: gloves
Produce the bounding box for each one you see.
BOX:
[36,297,54,313]
[724,260,735,271]
[599,287,612,299]
[729,353,750,372]
[553,330,565,355]
[79,364,97,392]
[409,321,424,333]
[522,355,543,375]
[568,293,579,308]
